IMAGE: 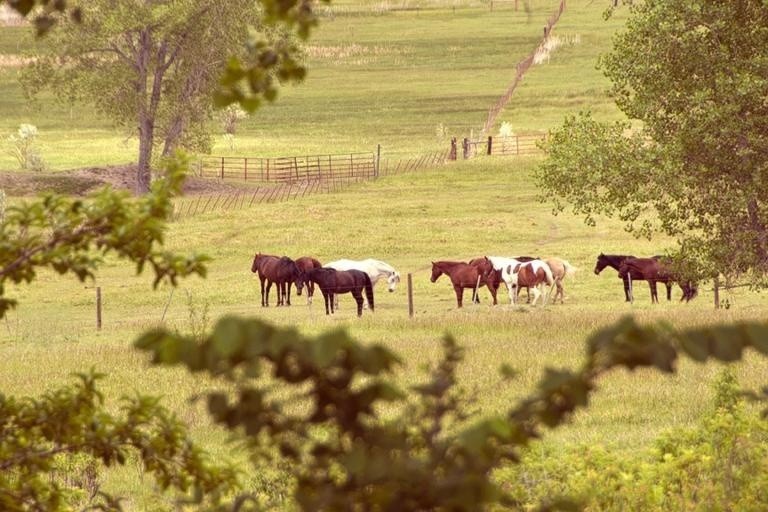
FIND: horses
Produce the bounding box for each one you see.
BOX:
[470,254,576,307]
[250,251,321,308]
[295,267,375,318]
[594,253,697,304]
[322,257,401,311]
[428,259,499,307]
